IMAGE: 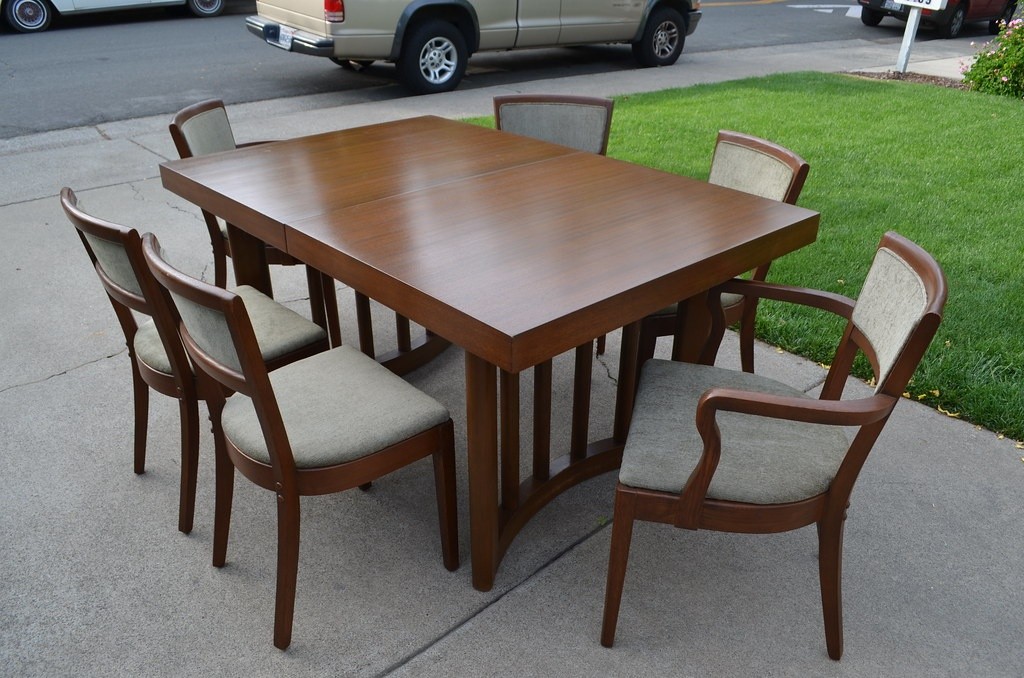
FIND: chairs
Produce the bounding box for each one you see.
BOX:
[596,129,810,374]
[601,227,948,663]
[142,232,460,651]
[59,188,330,537]
[169,98,327,330]
[494,94,616,354]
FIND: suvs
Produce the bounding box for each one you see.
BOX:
[246,0,704,95]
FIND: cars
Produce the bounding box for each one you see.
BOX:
[0,0,227,34]
[857,0,1019,39]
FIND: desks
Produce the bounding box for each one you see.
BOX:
[157,114,820,591]
